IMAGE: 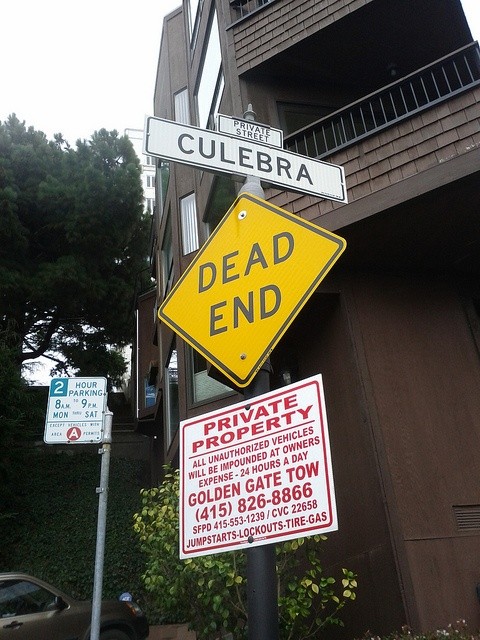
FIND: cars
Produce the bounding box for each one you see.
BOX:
[0,572,149,640]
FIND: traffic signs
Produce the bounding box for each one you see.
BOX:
[142,114,350,205]
